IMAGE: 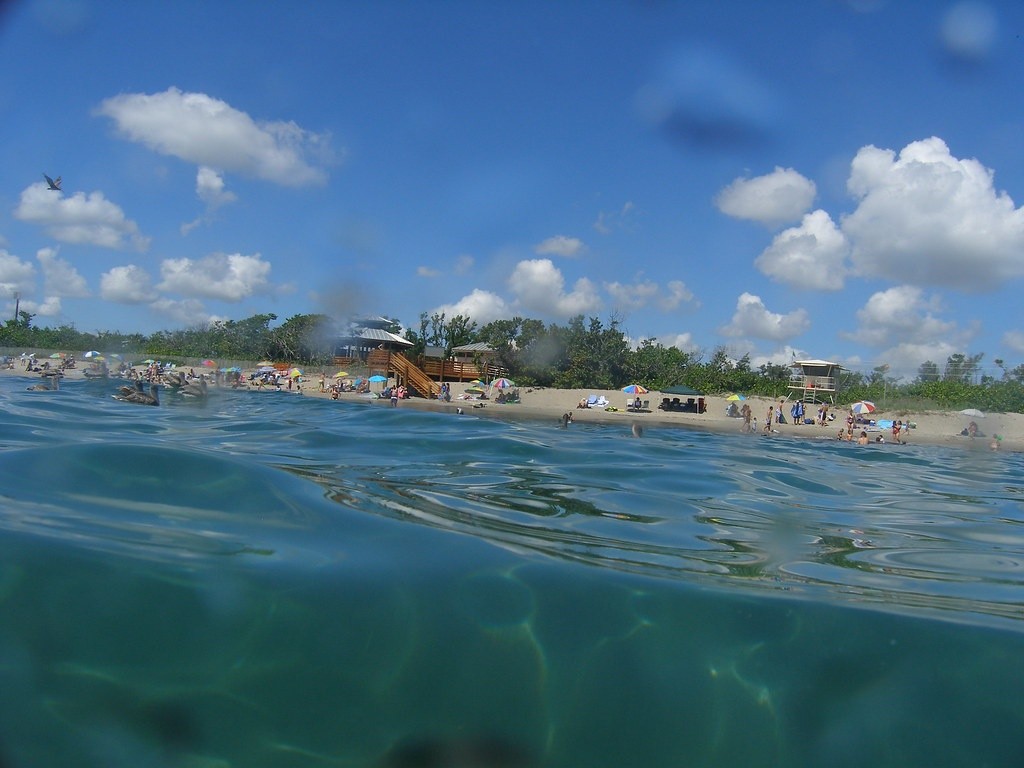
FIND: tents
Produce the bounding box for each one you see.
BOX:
[657,384,705,414]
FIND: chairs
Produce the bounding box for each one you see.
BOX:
[588,393,709,413]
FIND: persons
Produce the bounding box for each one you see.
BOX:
[0,352,1000,452]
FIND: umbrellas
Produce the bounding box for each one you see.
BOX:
[470,380,484,387]
[727,393,746,401]
[83,350,100,358]
[288,368,305,377]
[49,352,66,359]
[143,359,154,363]
[110,354,123,362]
[961,409,986,418]
[369,376,387,383]
[202,360,216,366]
[94,357,105,361]
[256,361,274,366]
[851,400,874,413]
[491,378,514,388]
[332,372,349,378]
[464,387,485,394]
[621,384,647,411]
